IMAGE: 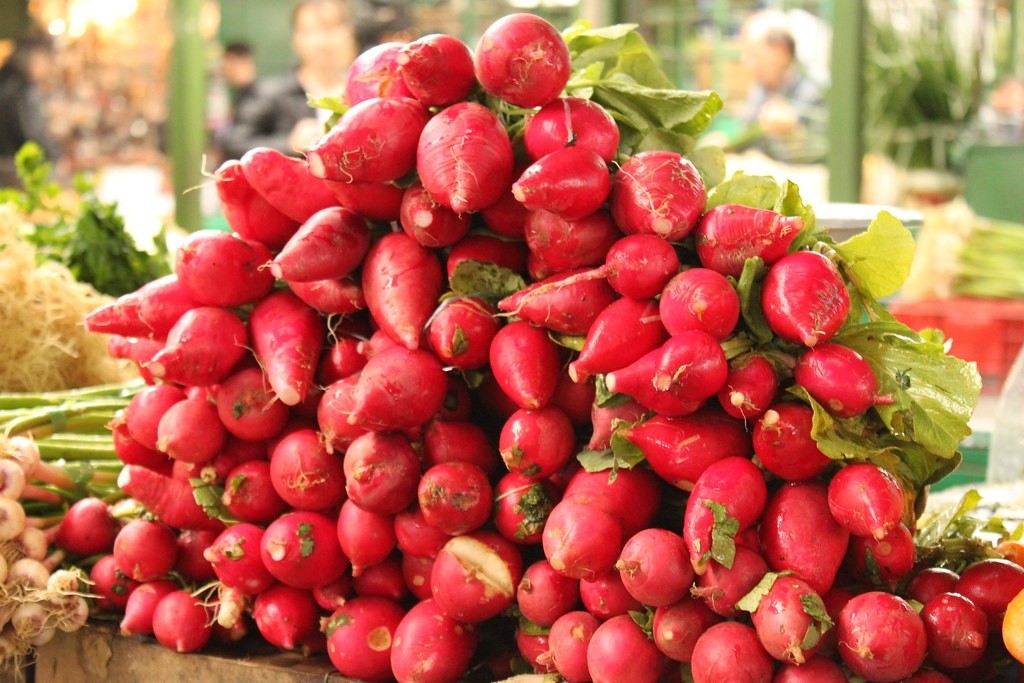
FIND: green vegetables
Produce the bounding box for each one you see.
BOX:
[0,140,175,301]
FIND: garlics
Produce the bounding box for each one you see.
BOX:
[1,435,89,680]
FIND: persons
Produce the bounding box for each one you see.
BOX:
[725,27,829,206]
[222,0,364,172]
[202,40,263,172]
